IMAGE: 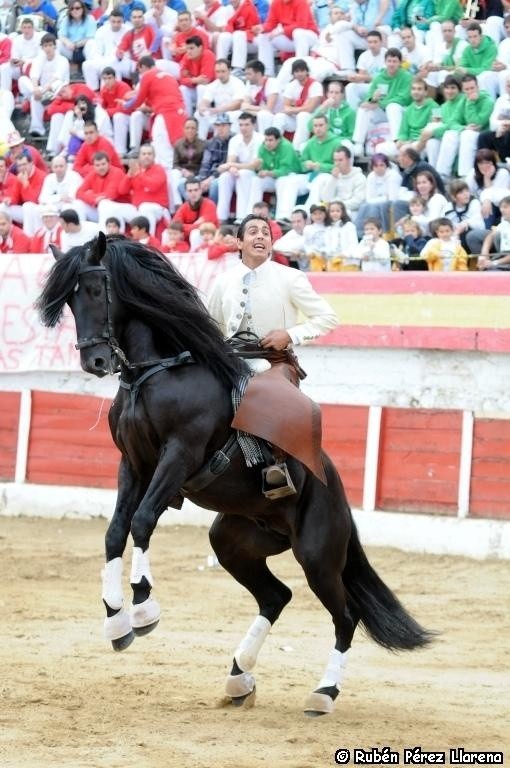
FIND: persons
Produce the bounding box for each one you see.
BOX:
[196,211,340,490]
[0,0,510,275]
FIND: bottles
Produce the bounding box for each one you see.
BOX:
[397,226,404,240]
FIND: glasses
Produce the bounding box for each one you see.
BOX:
[70,7,82,10]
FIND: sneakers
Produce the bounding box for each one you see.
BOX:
[230,68,247,81]
[125,149,139,158]
[333,70,357,77]
[41,92,55,105]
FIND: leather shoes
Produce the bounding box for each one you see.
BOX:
[265,464,286,488]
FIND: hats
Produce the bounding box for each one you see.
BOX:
[39,202,61,217]
[8,130,26,149]
[212,112,232,125]
[311,201,326,212]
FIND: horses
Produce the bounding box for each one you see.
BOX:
[30,230,441,717]
